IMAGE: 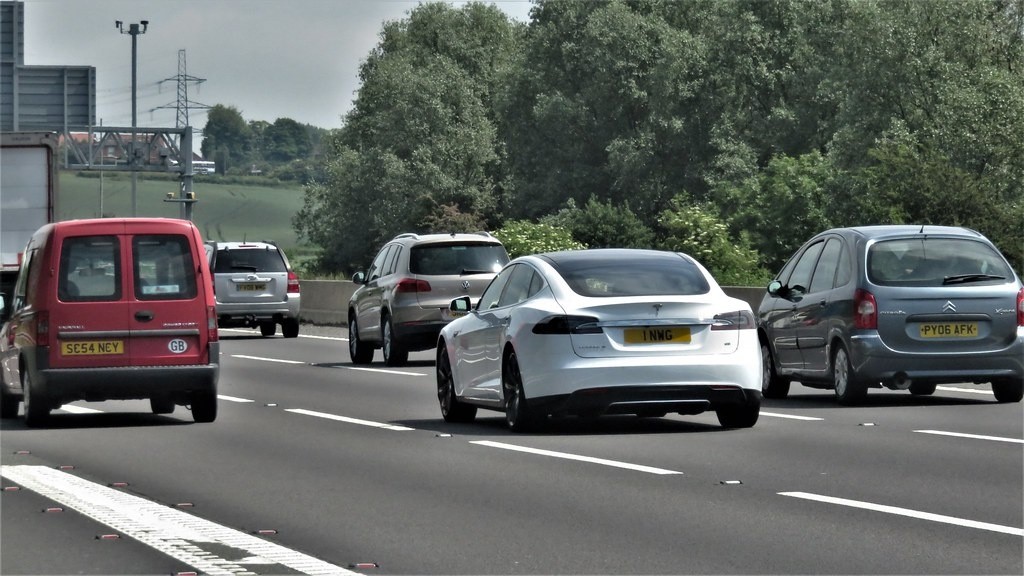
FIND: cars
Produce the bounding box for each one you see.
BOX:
[755,225,1024,403]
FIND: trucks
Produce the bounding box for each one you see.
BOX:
[0,130,60,322]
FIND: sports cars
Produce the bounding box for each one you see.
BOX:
[434,248,762,435]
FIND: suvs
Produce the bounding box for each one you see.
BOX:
[346,231,516,367]
[202,240,301,339]
[0,216,219,427]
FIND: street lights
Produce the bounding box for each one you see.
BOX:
[113,18,149,217]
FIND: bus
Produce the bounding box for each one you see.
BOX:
[171,160,216,176]
